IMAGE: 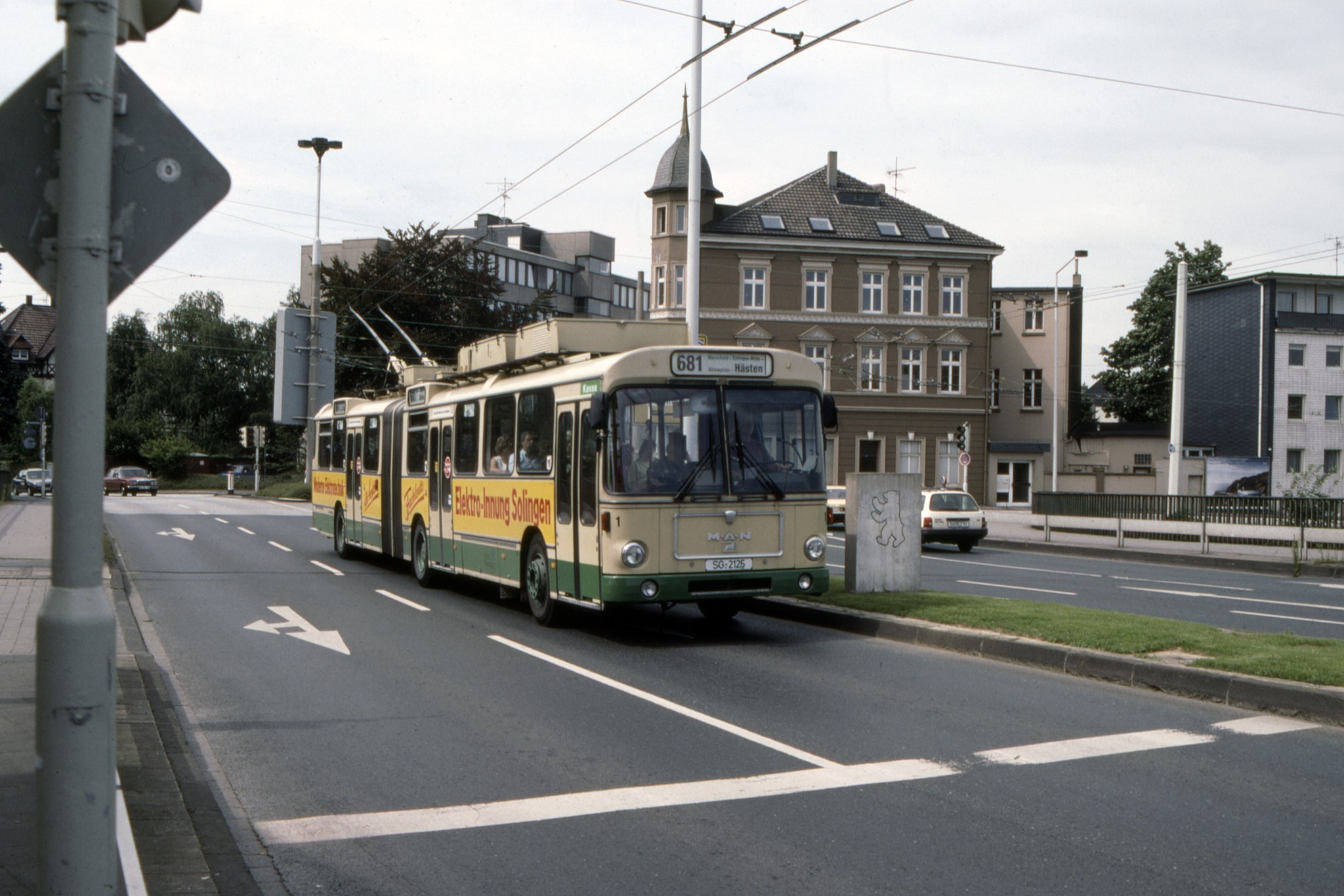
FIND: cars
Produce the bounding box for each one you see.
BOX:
[825,485,846,526]
[12,468,52,496]
[103,467,160,497]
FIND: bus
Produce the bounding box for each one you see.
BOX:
[311,317,841,627]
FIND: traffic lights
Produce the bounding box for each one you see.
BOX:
[240,426,255,448]
[42,421,52,449]
[259,427,269,450]
[956,425,967,450]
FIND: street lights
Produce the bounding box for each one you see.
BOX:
[1052,249,1088,493]
[293,138,345,488]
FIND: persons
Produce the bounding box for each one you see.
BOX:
[614,444,635,487]
[489,435,516,473]
[647,432,696,493]
[627,437,661,493]
[509,430,546,472]
[722,408,777,475]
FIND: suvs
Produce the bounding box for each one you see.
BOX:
[919,487,989,552]
